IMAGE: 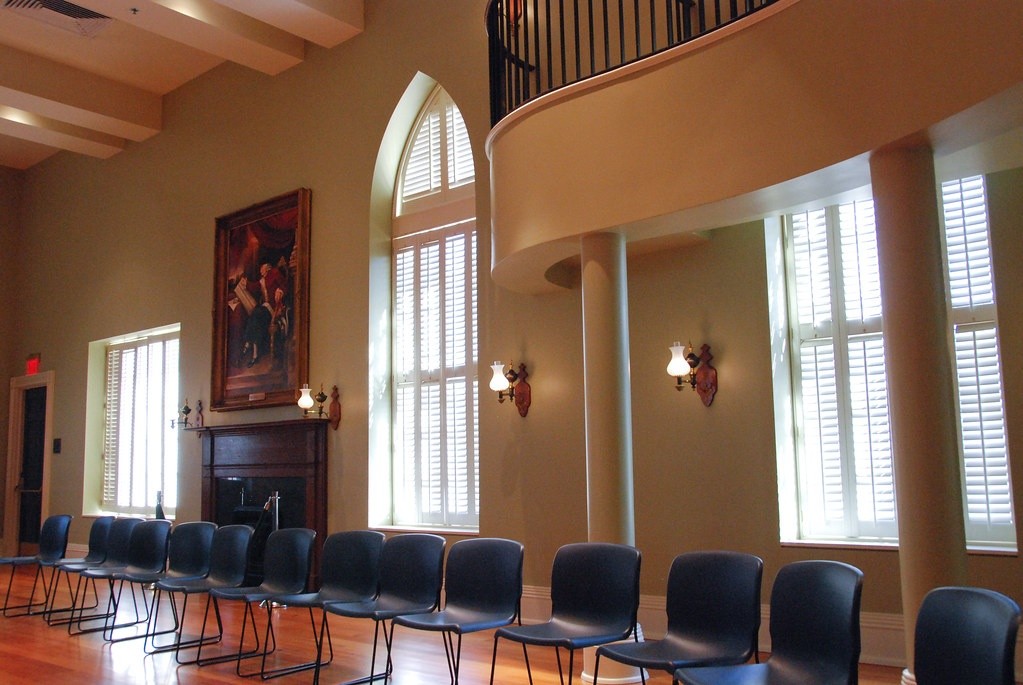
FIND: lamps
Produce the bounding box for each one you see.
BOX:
[164,398,204,438]
[667,340,719,408]
[297,383,341,431]
[489,359,531,417]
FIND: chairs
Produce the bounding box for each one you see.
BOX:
[196,530,322,678]
[383,539,533,685]
[110,522,223,650]
[27,516,118,623]
[143,523,255,664]
[0,514,74,619]
[488,543,646,685]
[671,560,865,684]
[261,529,392,685]
[914,587,1021,685]
[593,550,764,685]
[66,520,176,643]
[45,518,149,636]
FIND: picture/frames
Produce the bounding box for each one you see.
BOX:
[208,187,312,413]
